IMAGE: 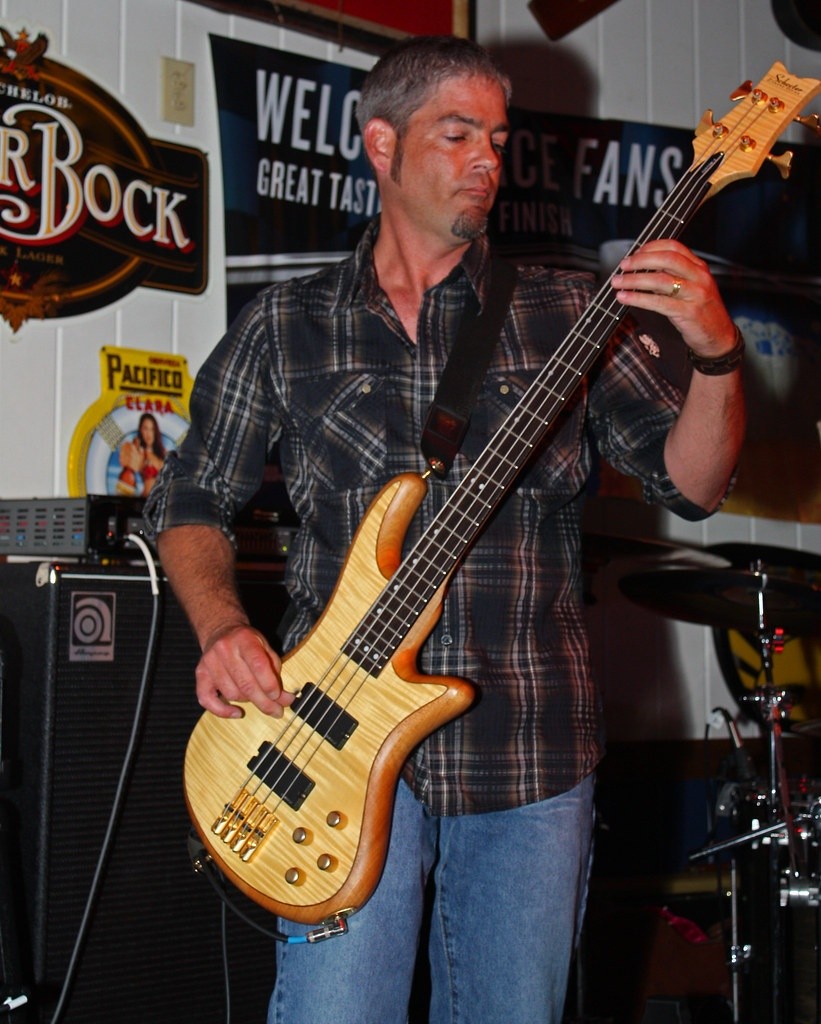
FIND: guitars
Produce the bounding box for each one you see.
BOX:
[183,61,819,925]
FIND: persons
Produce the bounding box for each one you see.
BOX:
[119,411,167,497]
[141,34,747,1024]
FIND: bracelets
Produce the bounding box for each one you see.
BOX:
[684,323,745,376]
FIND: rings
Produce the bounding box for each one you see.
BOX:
[668,276,681,298]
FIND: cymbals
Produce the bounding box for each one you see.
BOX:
[577,529,819,643]
